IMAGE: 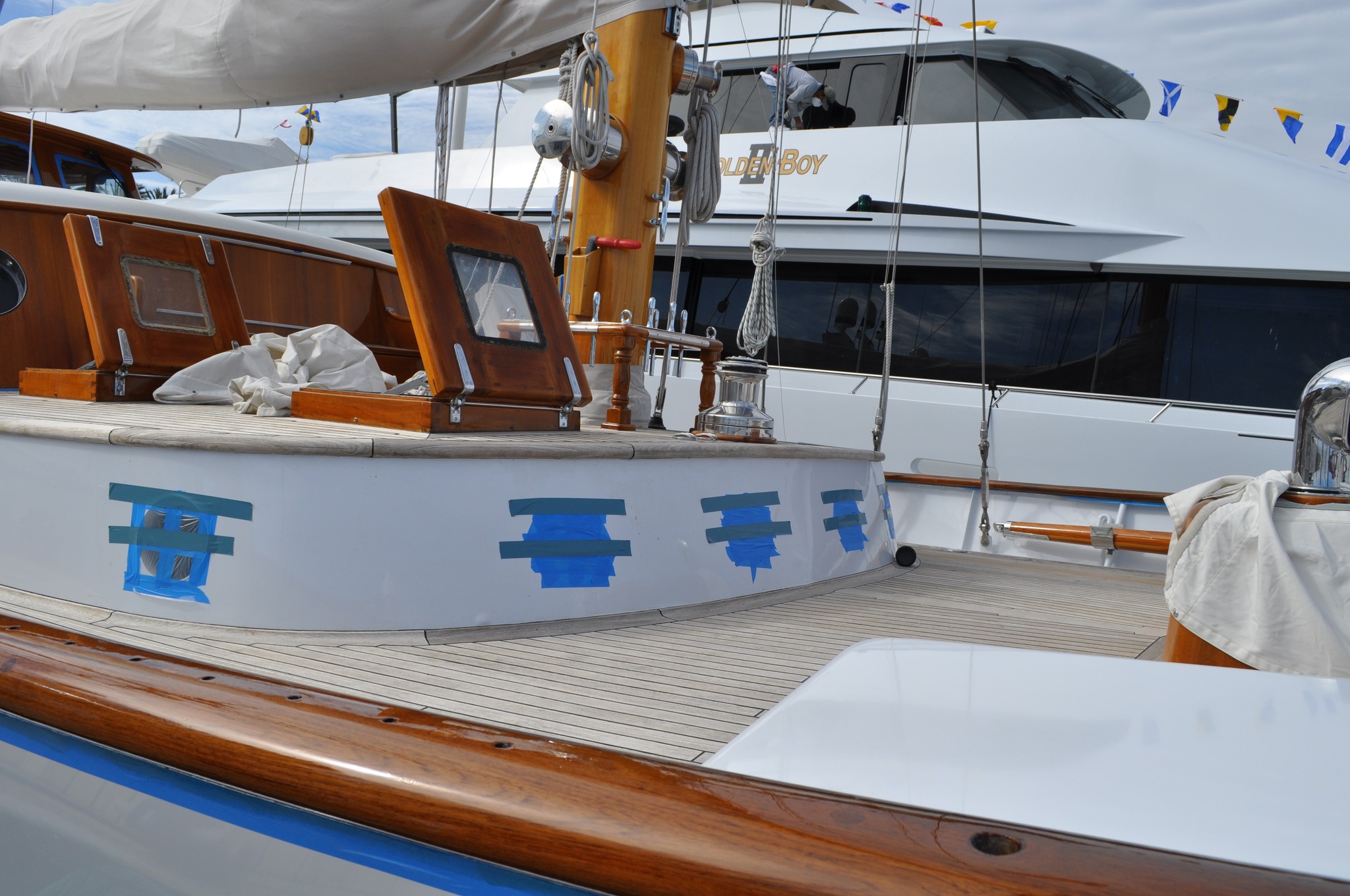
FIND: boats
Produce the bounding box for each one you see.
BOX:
[0,0,1350,896]
[193,0,1350,534]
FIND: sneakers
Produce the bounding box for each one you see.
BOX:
[768,124,791,132]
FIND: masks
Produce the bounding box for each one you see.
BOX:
[812,97,823,107]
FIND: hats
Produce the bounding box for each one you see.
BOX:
[822,86,836,111]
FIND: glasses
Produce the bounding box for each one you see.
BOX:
[823,93,831,106]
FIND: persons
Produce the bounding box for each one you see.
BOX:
[763,63,836,131]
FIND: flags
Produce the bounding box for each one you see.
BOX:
[1215,93,1240,131]
[1325,122,1350,165]
[296,105,321,123]
[275,119,292,128]
[914,13,942,26]
[959,20,997,30]
[1272,107,1303,144]
[1158,78,1183,117]
[875,2,910,14]
